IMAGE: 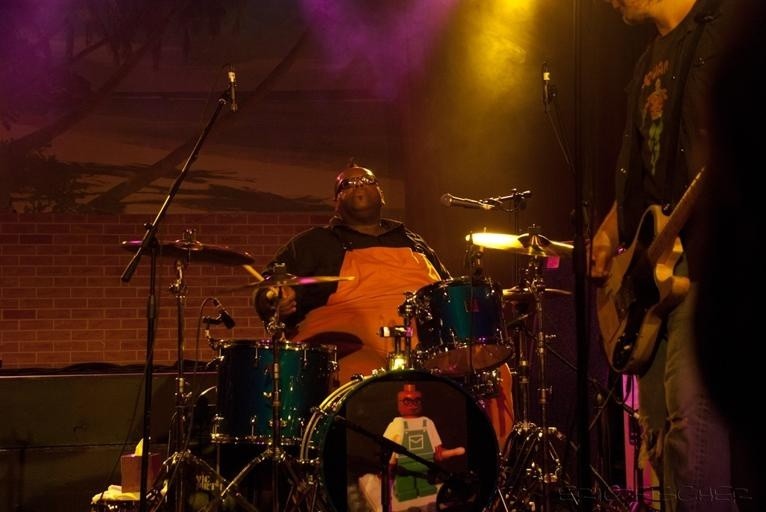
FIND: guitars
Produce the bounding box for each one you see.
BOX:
[597,166,708,375]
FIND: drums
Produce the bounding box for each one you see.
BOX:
[414,275,513,380]
[209,338,339,446]
[295,368,500,512]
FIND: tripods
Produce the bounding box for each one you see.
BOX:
[144,345,256,511]
[198,314,320,511]
[498,278,628,512]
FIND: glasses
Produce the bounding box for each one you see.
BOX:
[398,397,424,407]
[335,175,379,197]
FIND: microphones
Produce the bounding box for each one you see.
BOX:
[212,298,236,331]
[436,190,491,210]
[542,59,556,104]
[225,70,244,116]
[426,459,475,498]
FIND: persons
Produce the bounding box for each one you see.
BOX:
[252,162,453,404]
[383,382,464,502]
[592,1,766,510]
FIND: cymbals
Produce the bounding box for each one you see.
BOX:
[465,233,576,258]
[218,277,353,291]
[121,240,256,266]
[502,286,571,298]
